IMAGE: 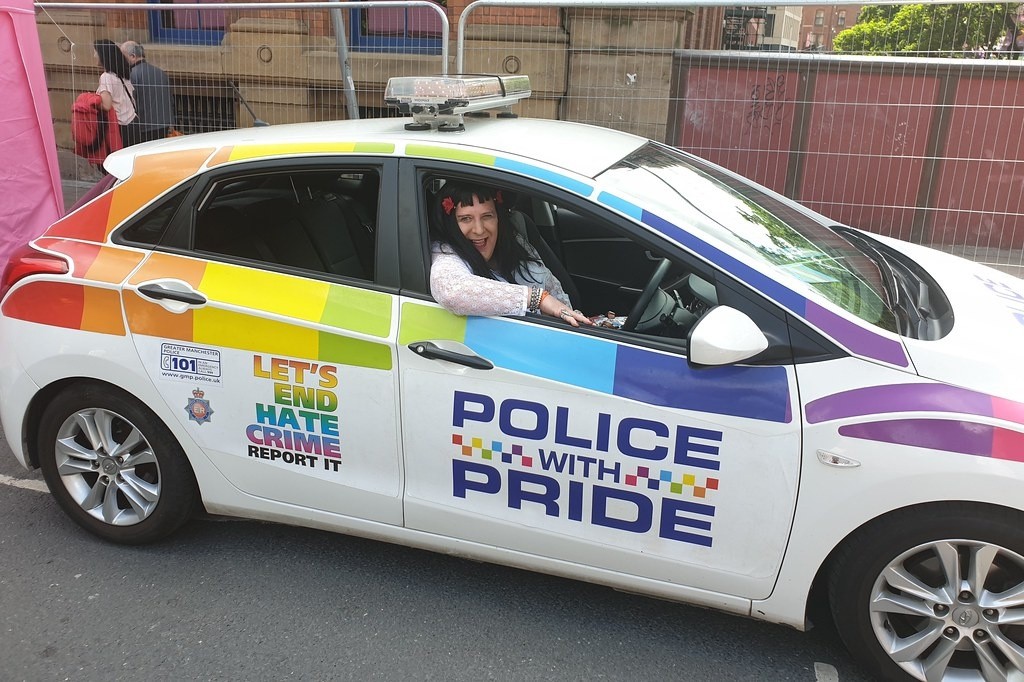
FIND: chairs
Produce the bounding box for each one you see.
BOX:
[514,209,584,312]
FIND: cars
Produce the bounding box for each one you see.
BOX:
[1,72,1024,681]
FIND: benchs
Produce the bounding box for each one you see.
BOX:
[137,187,376,282]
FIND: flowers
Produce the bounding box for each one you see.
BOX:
[442,197,453,214]
[496,189,504,204]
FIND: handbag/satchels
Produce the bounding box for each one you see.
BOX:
[168,127,184,138]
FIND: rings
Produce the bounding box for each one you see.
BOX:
[560,310,573,319]
[574,309,583,316]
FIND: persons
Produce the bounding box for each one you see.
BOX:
[429,178,597,328]
[93,39,137,176]
[120,41,176,141]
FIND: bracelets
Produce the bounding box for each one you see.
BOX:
[530,286,550,311]
